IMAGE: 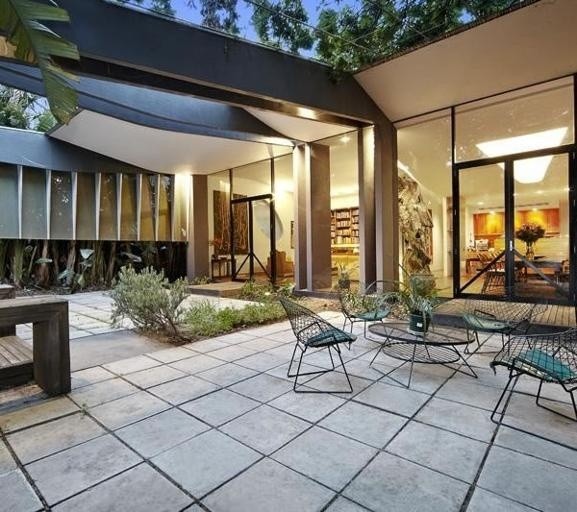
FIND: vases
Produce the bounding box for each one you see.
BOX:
[525,241,534,260]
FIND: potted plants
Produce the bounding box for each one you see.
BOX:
[335,261,360,289]
[362,261,484,338]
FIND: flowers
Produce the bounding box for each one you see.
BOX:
[516,224,545,244]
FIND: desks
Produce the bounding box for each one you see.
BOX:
[502,257,563,297]
[211,259,237,280]
[367,322,476,389]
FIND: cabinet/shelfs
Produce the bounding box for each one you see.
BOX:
[473,208,560,237]
[331,206,360,244]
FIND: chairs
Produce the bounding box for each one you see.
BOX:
[477,250,522,294]
[278,297,357,394]
[337,276,395,348]
[462,273,548,354]
[488,323,577,450]
